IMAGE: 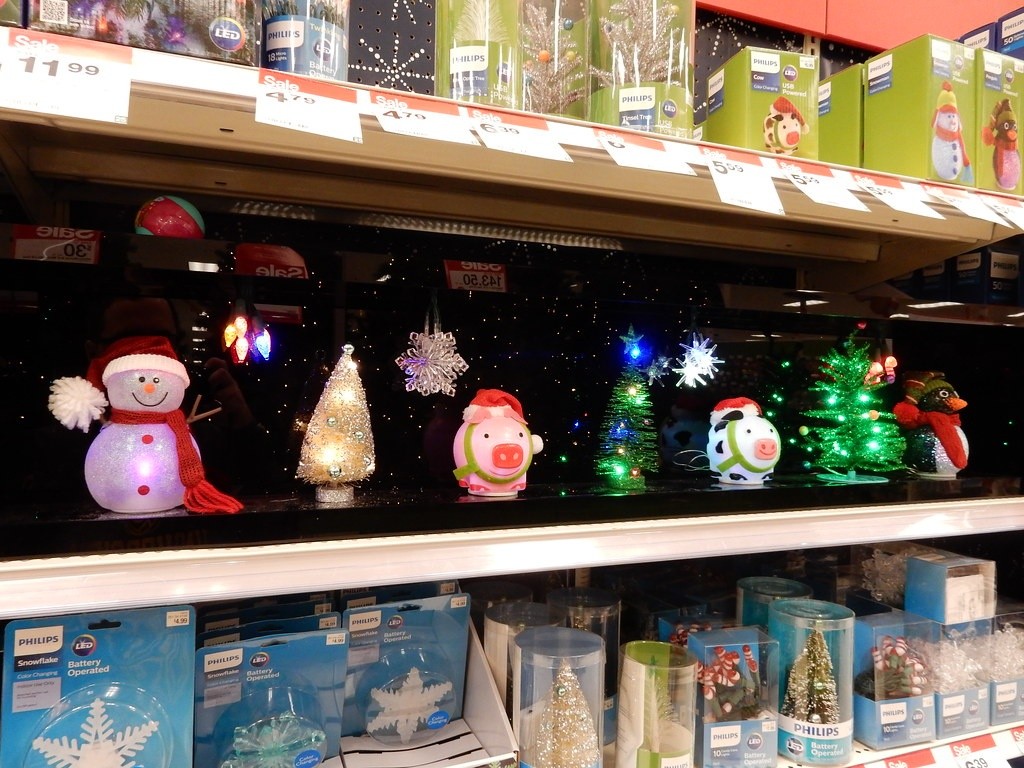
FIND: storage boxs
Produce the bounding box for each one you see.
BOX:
[309,584,521,768]
[814,543,1024,751]
[696,7,1024,195]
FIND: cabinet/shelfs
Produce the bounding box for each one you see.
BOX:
[0,27,1024,768]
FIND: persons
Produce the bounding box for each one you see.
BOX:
[82,292,265,469]
